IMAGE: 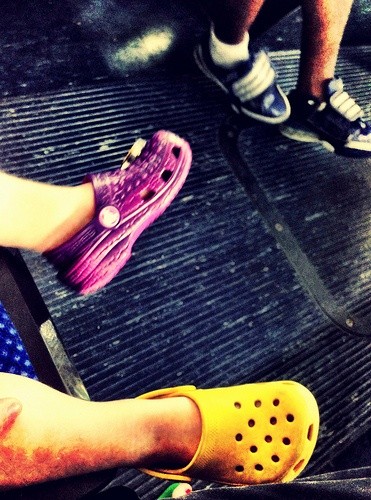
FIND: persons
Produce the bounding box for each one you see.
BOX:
[0,129,320,489]
[193,0,371,161]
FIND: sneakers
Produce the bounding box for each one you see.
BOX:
[193,31,291,126]
[279,75,371,159]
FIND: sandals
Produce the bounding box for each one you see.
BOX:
[132,380,319,486]
[42,129,193,295]
